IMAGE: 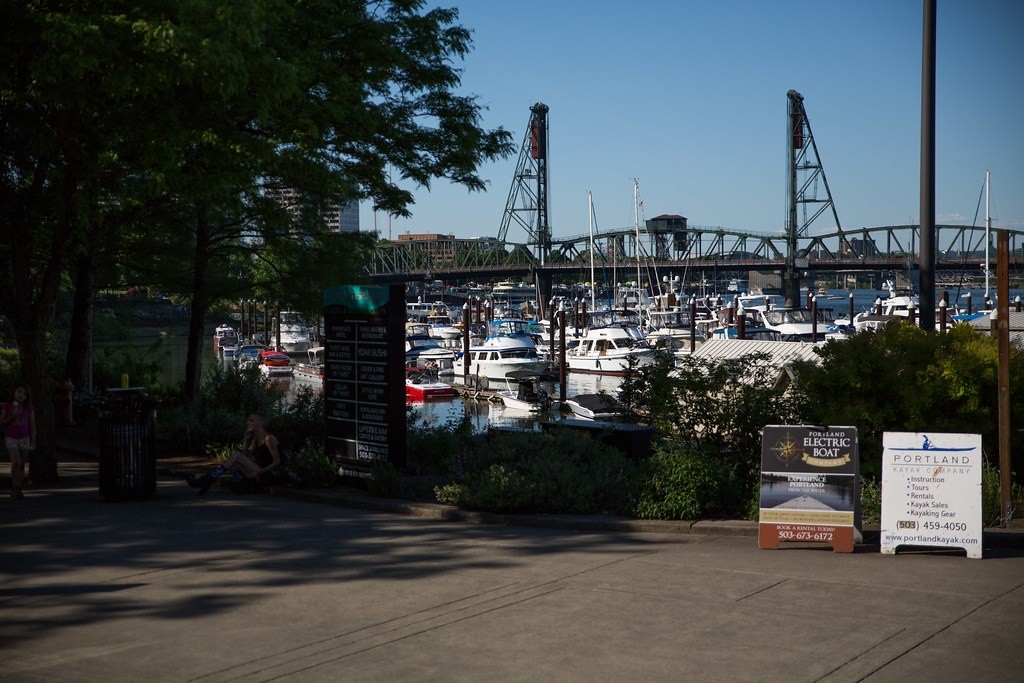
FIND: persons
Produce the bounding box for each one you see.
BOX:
[186,411,287,495]
[0,381,36,500]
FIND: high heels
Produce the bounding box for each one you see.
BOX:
[238,479,259,495]
[185,475,211,495]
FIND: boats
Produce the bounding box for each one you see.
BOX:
[403,178,958,382]
[292,347,325,383]
[728,277,749,292]
[406,359,454,406]
[271,304,312,355]
[259,349,292,376]
[498,368,560,411]
[213,324,268,373]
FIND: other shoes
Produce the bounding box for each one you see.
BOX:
[10,488,24,500]
